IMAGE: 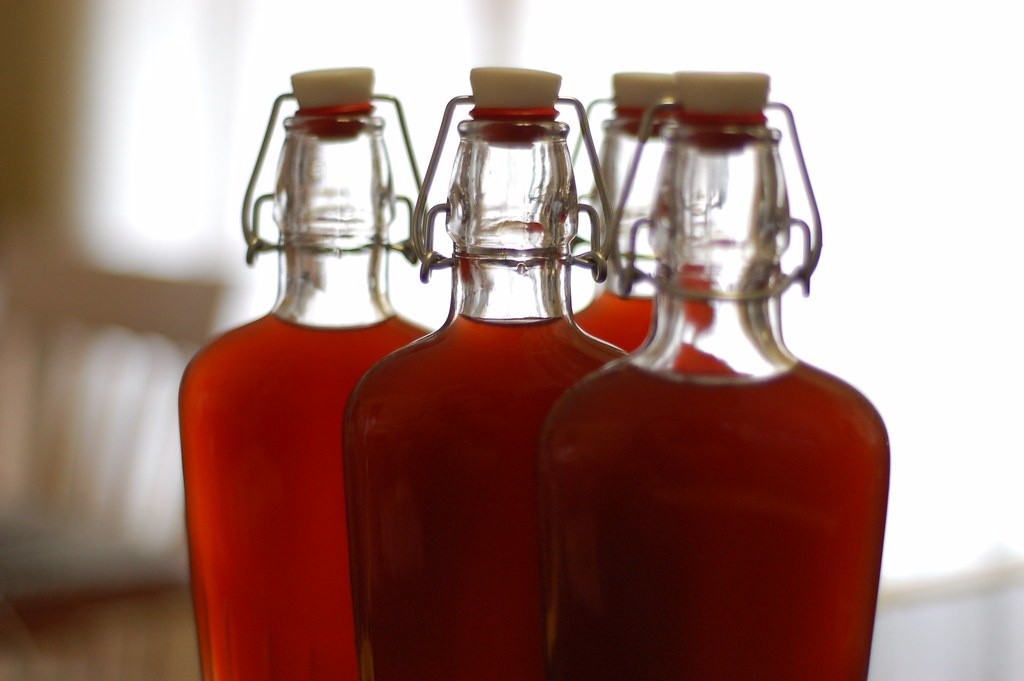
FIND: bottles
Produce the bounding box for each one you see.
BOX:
[348,68,628,681]
[562,71,673,357]
[177,68,434,681]
[538,71,890,681]
